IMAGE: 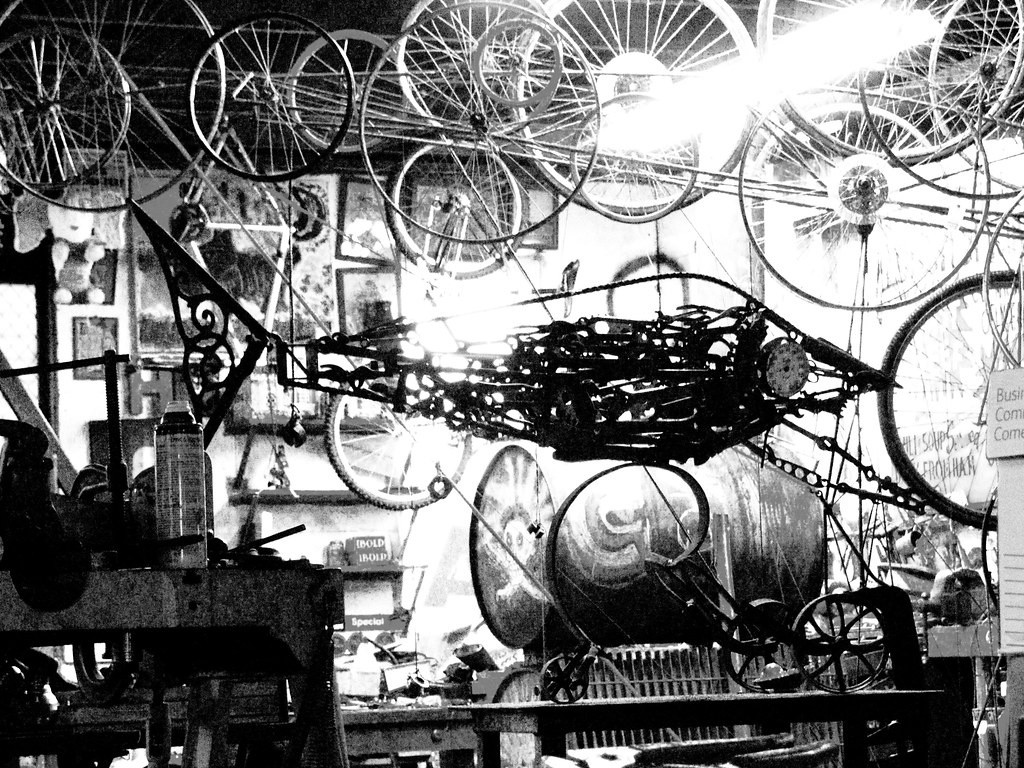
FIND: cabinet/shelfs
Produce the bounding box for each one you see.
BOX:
[221,415,428,636]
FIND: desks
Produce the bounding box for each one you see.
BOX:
[341,704,478,768]
[447,690,943,768]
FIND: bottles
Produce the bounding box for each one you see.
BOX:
[328,540,348,566]
[154,400,207,569]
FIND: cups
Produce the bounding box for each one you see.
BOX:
[892,527,921,557]
[456,644,499,672]
[406,674,429,698]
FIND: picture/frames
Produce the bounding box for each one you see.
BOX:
[73,316,119,381]
[335,267,403,333]
[56,246,119,305]
[127,167,231,355]
[404,174,504,261]
[504,180,559,251]
[334,175,400,265]
